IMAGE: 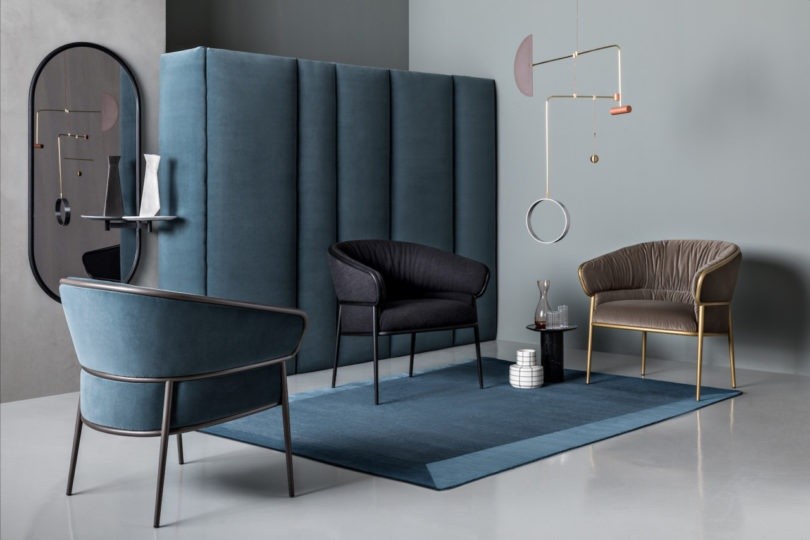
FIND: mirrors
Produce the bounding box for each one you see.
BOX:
[29,42,141,304]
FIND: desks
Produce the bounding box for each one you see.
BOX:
[526,323,579,384]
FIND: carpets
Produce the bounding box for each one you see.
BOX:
[194,356,745,490]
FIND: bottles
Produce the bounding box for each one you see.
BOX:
[535,279,552,327]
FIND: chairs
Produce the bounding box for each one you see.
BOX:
[579,239,741,402]
[327,239,490,406]
[59,275,307,527]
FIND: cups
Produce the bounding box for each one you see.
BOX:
[546,305,568,329]
